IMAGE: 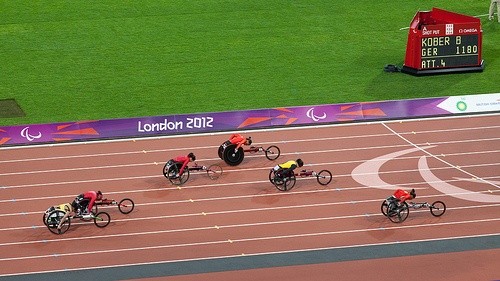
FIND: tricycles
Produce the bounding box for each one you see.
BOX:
[268,166,333,192]
[163,158,224,187]
[218,139,281,165]
[379,195,446,222]
[43,206,111,236]
[73,198,135,216]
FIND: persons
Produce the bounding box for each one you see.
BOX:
[392,189,416,207]
[489,0,500,24]
[226,134,252,153]
[71,191,103,215]
[168,153,196,177]
[49,203,78,230]
[274,159,303,177]
